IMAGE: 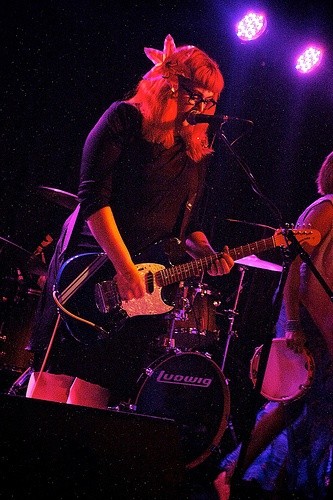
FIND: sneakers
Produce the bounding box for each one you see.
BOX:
[213,471,230,500]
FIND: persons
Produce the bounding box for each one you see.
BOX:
[23,44,237,412]
[212,150,333,500]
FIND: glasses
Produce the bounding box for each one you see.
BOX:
[182,85,219,109]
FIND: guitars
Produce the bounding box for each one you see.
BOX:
[53,222,321,346]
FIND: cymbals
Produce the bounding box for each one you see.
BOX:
[35,182,79,208]
[232,254,283,272]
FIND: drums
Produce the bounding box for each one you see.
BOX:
[123,345,230,470]
[165,283,221,347]
[249,338,314,404]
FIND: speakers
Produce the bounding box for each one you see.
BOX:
[0,393,198,500]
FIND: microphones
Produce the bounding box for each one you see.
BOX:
[184,110,253,126]
